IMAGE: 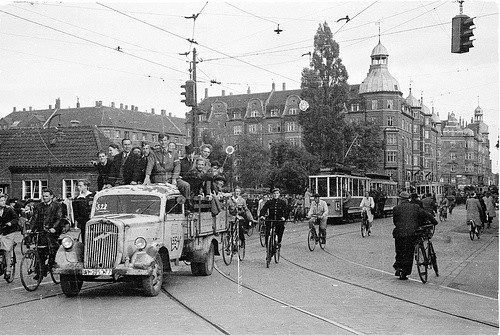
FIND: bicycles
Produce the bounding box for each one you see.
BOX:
[18,229,60,292]
[468,221,481,240]
[308,217,327,252]
[223,219,246,266]
[413,222,440,285]
[361,210,373,238]
[264,218,282,268]
[440,210,448,221]
[0,244,15,281]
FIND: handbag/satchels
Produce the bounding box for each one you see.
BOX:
[371,208,375,214]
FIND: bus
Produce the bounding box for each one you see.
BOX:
[413,182,448,208]
[306,163,400,226]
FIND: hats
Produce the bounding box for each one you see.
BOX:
[142,142,148,148]
[270,186,280,193]
[185,144,197,154]
[411,194,419,197]
[311,194,322,197]
[399,192,411,199]
[213,174,227,182]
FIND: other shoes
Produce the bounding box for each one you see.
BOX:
[400,275,406,279]
[395,269,402,276]
[187,199,194,210]
[4,271,11,279]
[241,242,245,248]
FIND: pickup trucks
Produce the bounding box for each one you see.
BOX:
[58,181,230,296]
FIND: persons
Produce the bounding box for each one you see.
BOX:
[422,194,426,198]
[227,185,289,251]
[370,186,387,215]
[289,194,304,220]
[409,193,423,208]
[466,190,499,233]
[303,187,312,214]
[72,180,95,244]
[420,193,437,217]
[360,191,375,233]
[306,193,328,243]
[437,188,467,219]
[91,133,231,214]
[392,191,438,280]
[0,188,62,279]
[343,191,351,222]
[432,193,438,213]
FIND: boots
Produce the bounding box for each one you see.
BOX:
[35,246,47,280]
[311,228,317,239]
[49,245,58,269]
[322,229,326,244]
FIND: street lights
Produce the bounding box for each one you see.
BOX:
[47,113,62,197]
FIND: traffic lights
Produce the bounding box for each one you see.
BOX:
[451,15,476,54]
[179,79,196,107]
